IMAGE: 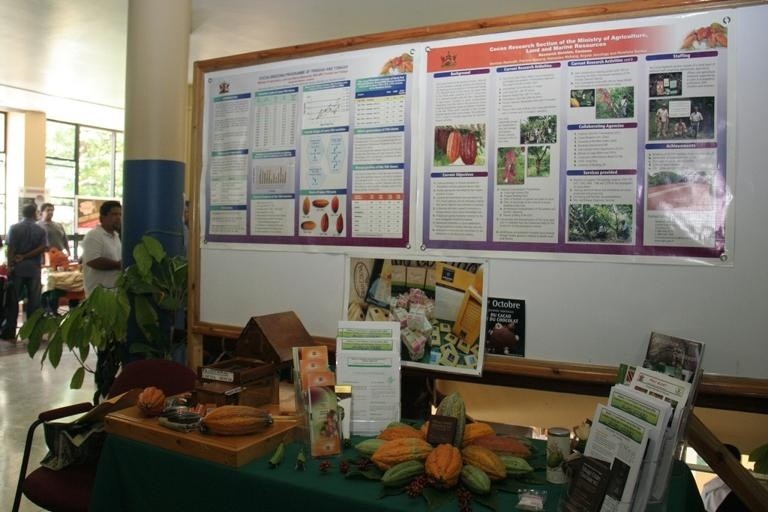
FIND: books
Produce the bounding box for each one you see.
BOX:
[561,330,705,512]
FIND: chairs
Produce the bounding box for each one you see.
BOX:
[11,359,196,512]
[43,251,86,311]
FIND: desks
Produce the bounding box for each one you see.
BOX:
[92,402,741,512]
[40,267,86,316]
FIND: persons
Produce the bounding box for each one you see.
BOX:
[35,203,70,265]
[81,201,124,399]
[0,203,48,341]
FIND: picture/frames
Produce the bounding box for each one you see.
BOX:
[74,196,123,235]
[342,253,488,377]
